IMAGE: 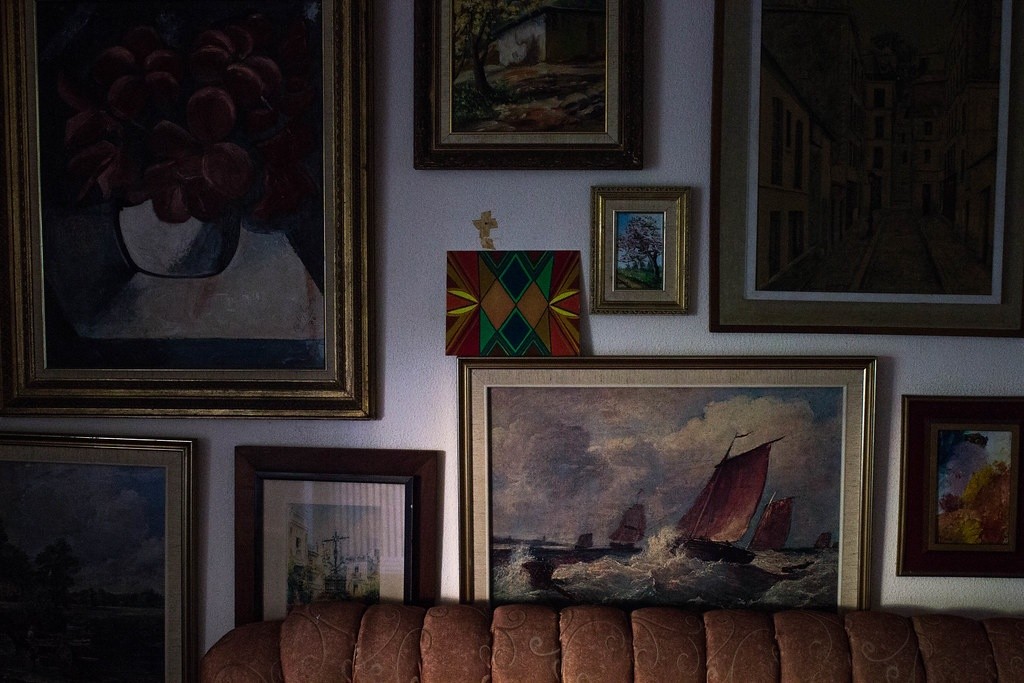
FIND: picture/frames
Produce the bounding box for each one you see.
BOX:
[588,186,695,315]
[413,0,644,170]
[0,431,197,683]
[458,355,883,610]
[234,445,439,629]
[708,0,1024,337]
[0,0,377,421]
[896,394,1024,578]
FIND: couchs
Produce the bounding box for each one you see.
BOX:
[199,606,1024,683]
